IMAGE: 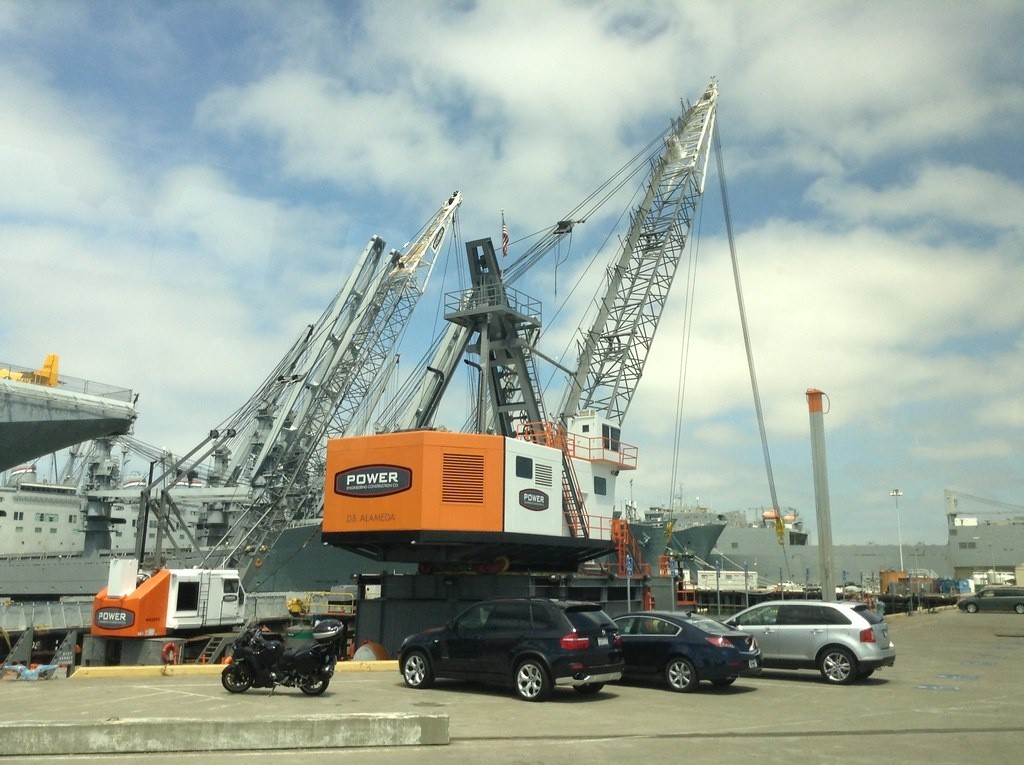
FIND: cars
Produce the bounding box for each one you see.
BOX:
[611,610,763,693]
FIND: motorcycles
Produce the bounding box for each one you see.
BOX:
[222,615,344,696]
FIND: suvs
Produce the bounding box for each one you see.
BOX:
[398,597,625,703]
[720,599,896,685]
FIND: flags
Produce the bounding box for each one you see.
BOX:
[502,214,509,256]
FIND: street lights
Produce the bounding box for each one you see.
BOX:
[891,488,906,572]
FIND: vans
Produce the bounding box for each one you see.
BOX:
[956,586,1024,615]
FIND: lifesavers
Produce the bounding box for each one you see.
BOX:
[163,643,179,661]
